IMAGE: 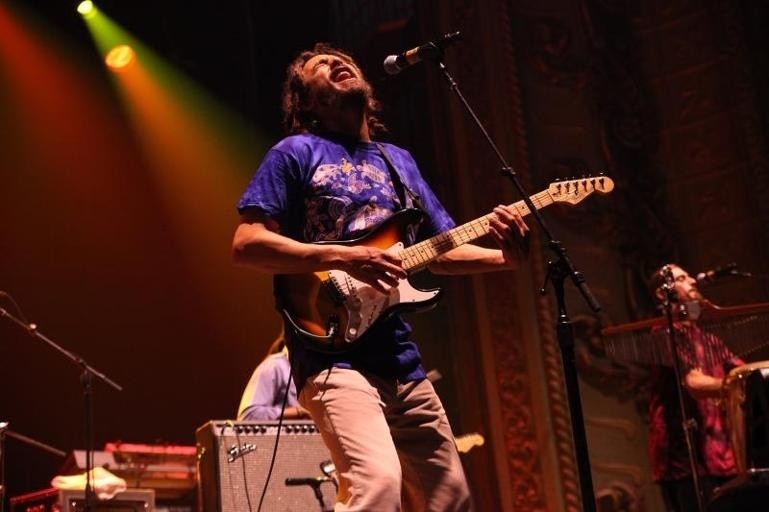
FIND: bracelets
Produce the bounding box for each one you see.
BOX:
[295,405,303,418]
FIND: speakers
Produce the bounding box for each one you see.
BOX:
[196,421,340,512]
[743,366,769,471]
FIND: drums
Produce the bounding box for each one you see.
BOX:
[725,357,769,481]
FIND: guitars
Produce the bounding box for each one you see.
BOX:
[272,172,614,344]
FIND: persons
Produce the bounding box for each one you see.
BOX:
[648,265,769,510]
[236,323,313,420]
[232,45,532,512]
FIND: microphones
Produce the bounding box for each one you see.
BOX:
[696,262,738,287]
[283,475,331,486]
[383,31,462,76]
